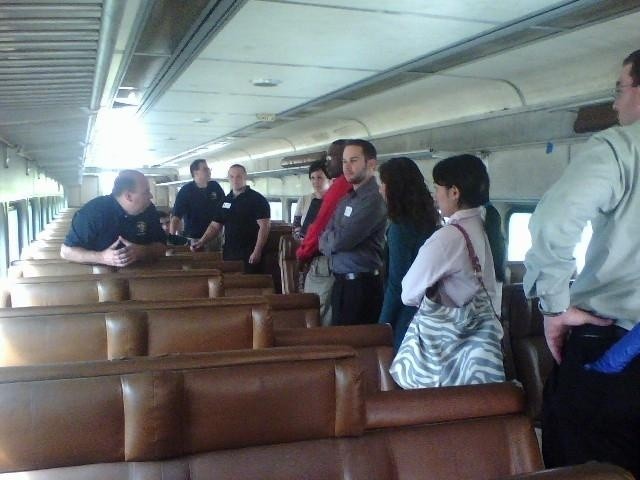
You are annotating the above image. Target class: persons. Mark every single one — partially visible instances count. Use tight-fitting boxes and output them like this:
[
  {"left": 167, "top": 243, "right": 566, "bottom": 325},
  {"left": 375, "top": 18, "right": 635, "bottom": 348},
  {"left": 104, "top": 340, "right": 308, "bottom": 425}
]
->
[
  {"left": 169, "top": 158, "right": 227, "bottom": 254},
  {"left": 522, "top": 49, "right": 639, "bottom": 479},
  {"left": 376, "top": 156, "right": 445, "bottom": 366},
  {"left": 291, "top": 138, "right": 391, "bottom": 328},
  {"left": 399, "top": 153, "right": 507, "bottom": 358},
  {"left": 190, "top": 164, "right": 271, "bottom": 274},
  {"left": 59, "top": 169, "right": 167, "bottom": 268},
  {"left": 155, "top": 209, "right": 170, "bottom": 238}
]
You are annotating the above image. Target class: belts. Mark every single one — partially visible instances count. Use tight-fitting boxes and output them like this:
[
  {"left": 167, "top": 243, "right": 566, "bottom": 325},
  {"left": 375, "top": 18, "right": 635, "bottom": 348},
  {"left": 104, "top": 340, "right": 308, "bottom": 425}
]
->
[{"left": 333, "top": 270, "right": 380, "bottom": 280}]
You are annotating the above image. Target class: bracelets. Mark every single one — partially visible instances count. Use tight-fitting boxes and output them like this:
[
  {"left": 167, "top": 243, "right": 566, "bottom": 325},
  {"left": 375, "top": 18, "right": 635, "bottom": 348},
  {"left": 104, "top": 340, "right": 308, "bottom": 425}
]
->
[{"left": 537, "top": 300, "right": 568, "bottom": 318}]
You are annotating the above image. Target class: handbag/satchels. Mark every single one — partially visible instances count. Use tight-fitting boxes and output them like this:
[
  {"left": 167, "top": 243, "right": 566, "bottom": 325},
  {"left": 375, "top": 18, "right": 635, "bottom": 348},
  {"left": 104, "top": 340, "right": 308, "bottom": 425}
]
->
[{"left": 389, "top": 224, "right": 505, "bottom": 390}]
